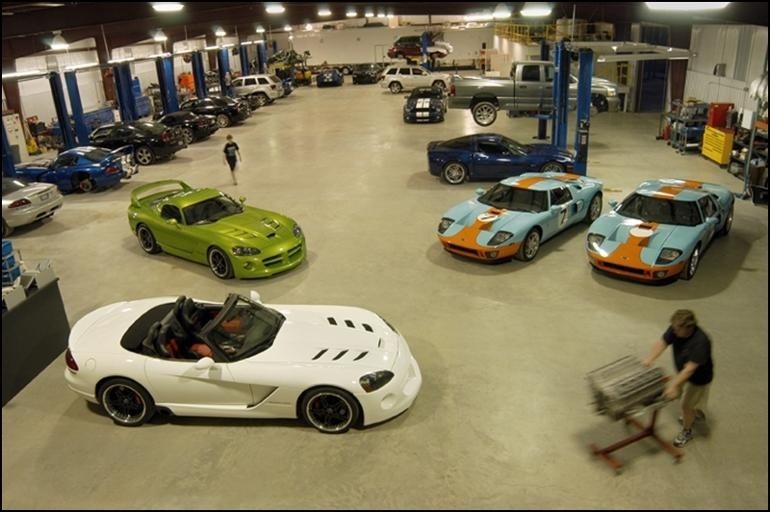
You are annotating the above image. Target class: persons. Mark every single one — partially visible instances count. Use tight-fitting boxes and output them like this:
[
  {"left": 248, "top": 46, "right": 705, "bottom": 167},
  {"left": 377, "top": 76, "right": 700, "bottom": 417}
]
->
[
  {"left": 249, "top": 58, "right": 256, "bottom": 73},
  {"left": 224, "top": 71, "right": 234, "bottom": 98},
  {"left": 223, "top": 134, "right": 243, "bottom": 186},
  {"left": 642, "top": 308, "right": 714, "bottom": 447}
]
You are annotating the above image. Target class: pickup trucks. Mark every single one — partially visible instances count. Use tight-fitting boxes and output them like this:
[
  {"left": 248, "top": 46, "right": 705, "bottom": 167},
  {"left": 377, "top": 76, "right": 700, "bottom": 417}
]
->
[{"left": 450, "top": 60, "right": 621, "bottom": 128}]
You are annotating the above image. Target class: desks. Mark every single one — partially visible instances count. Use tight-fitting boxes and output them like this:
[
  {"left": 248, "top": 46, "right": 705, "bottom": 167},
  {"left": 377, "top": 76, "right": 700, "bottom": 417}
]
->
[{"left": 1, "top": 277, "right": 70, "bottom": 407}]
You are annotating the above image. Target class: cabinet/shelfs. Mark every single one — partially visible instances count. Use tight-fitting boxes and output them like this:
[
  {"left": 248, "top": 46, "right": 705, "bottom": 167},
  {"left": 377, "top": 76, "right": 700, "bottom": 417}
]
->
[
  {"left": 0, "top": 248, "right": 26, "bottom": 287},
  {"left": 727, "top": 123, "right": 768, "bottom": 183}
]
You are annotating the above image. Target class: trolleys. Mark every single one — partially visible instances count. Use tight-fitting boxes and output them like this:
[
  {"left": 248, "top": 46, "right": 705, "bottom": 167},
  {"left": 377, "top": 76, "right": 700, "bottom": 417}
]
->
[{"left": 591, "top": 377, "right": 683, "bottom": 475}]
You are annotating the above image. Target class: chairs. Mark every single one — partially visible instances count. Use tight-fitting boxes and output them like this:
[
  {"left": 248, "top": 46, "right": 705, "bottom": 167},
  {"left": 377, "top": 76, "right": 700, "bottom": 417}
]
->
[
  {"left": 147, "top": 323, "right": 194, "bottom": 359},
  {"left": 174, "top": 294, "right": 212, "bottom": 333}
]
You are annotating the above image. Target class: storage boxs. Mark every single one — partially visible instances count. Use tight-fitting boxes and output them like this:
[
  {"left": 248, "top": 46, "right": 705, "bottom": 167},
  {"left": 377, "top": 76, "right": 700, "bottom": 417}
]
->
[
  {"left": 1, "top": 282, "right": 27, "bottom": 311},
  {"left": 21, "top": 258, "right": 58, "bottom": 290},
  {"left": 1, "top": 237, "right": 19, "bottom": 282}
]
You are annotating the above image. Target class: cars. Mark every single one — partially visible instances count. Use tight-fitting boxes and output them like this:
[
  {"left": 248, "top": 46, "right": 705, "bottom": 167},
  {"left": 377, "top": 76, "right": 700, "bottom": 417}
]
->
[
  {"left": 382, "top": 65, "right": 450, "bottom": 94},
  {"left": 353, "top": 63, "right": 384, "bottom": 84},
  {"left": 231, "top": 74, "right": 285, "bottom": 108},
  {"left": 276, "top": 71, "right": 294, "bottom": 96},
  {"left": 403, "top": 87, "right": 447, "bottom": 124},
  {"left": 157, "top": 111, "right": 218, "bottom": 145},
  {"left": 180, "top": 96, "right": 250, "bottom": 127},
  {"left": 94, "top": 121, "right": 189, "bottom": 165},
  {"left": 388, "top": 35, "right": 453, "bottom": 60}
]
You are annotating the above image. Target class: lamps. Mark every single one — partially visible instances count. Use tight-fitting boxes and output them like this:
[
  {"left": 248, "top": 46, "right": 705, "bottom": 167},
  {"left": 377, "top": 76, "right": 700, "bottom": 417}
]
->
[
  {"left": 317, "top": 5, "right": 332, "bottom": 16},
  {"left": 387, "top": 10, "right": 394, "bottom": 18},
  {"left": 257, "top": 25, "right": 265, "bottom": 33},
  {"left": 154, "top": 28, "right": 168, "bottom": 42},
  {"left": 284, "top": 24, "right": 292, "bottom": 31},
  {"left": 346, "top": 6, "right": 357, "bottom": 18},
  {"left": 215, "top": 27, "right": 227, "bottom": 37},
  {"left": 464, "top": 1, "right": 553, "bottom": 22},
  {"left": 377, "top": 8, "right": 387, "bottom": 18},
  {"left": 51, "top": 30, "right": 70, "bottom": 51},
  {"left": 365, "top": 6, "right": 374, "bottom": 18}
]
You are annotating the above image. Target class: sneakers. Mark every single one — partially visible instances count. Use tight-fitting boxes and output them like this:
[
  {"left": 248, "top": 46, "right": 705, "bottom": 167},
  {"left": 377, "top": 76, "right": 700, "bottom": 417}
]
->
[
  {"left": 673, "top": 429, "right": 694, "bottom": 448},
  {"left": 678, "top": 410, "right": 706, "bottom": 425}
]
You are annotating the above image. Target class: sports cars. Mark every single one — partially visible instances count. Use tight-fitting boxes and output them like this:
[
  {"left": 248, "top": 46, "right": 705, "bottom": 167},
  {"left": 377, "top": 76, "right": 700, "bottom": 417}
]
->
[
  {"left": 436, "top": 171, "right": 602, "bottom": 267},
  {"left": 316, "top": 68, "right": 344, "bottom": 88},
  {"left": 14, "top": 146, "right": 128, "bottom": 194},
  {"left": 126, "top": 178, "right": 306, "bottom": 278},
  {"left": 584, "top": 177, "right": 734, "bottom": 283},
  {"left": 428, "top": 133, "right": 574, "bottom": 186},
  {"left": 64, "top": 290, "right": 422, "bottom": 434},
  {"left": 1, "top": 177, "right": 65, "bottom": 238}
]
